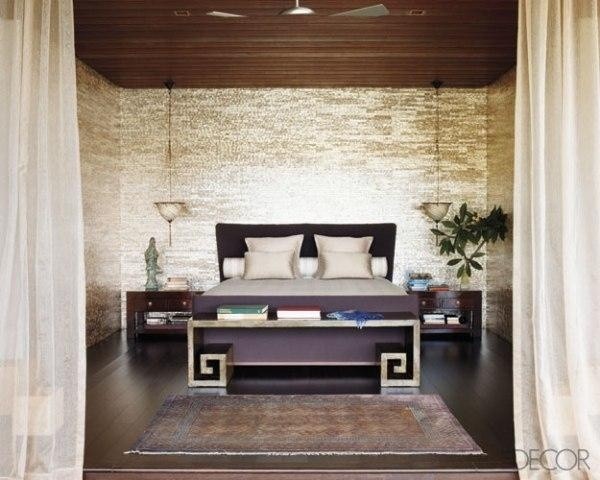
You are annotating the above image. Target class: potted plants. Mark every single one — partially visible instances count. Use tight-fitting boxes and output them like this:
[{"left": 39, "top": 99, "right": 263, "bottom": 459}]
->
[{"left": 429, "top": 203, "right": 510, "bottom": 290}]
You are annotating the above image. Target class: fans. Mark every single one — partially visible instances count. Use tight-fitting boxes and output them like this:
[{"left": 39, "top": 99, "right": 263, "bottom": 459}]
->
[{"left": 205, "top": 0, "right": 390, "bottom": 19}]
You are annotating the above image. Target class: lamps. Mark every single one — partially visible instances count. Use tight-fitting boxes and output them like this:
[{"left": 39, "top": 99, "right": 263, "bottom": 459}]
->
[
  {"left": 422, "top": 80, "right": 452, "bottom": 230},
  {"left": 155, "top": 80, "right": 185, "bottom": 245}
]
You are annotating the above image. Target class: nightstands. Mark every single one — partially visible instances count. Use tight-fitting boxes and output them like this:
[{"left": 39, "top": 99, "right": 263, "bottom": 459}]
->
[
  {"left": 126, "top": 291, "right": 205, "bottom": 340},
  {"left": 409, "top": 290, "right": 482, "bottom": 341}
]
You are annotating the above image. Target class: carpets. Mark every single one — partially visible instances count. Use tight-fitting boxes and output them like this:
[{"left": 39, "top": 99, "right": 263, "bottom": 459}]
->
[{"left": 123, "top": 394, "right": 488, "bottom": 455}]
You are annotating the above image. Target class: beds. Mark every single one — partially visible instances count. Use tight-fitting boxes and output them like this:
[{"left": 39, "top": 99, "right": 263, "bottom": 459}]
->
[{"left": 187, "top": 223, "right": 420, "bottom": 389}]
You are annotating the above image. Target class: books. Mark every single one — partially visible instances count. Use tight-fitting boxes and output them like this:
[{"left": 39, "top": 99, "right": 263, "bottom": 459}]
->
[
  {"left": 407, "top": 272, "right": 433, "bottom": 293},
  {"left": 428, "top": 283, "right": 449, "bottom": 292},
  {"left": 216, "top": 302, "right": 269, "bottom": 314},
  {"left": 445, "top": 314, "right": 459, "bottom": 321},
  {"left": 276, "top": 303, "right": 321, "bottom": 320},
  {"left": 418, "top": 310, "right": 446, "bottom": 320},
  {"left": 446, "top": 320, "right": 461, "bottom": 325},
  {"left": 144, "top": 312, "right": 190, "bottom": 326},
  {"left": 216, "top": 311, "right": 270, "bottom": 321},
  {"left": 420, "top": 319, "right": 447, "bottom": 325}
]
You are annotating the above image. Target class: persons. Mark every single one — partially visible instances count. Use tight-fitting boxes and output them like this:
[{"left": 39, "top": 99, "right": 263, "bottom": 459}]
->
[{"left": 144, "top": 237, "right": 163, "bottom": 291}]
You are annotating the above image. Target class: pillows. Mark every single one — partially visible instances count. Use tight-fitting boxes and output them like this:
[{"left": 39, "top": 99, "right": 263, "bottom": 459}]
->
[{"left": 222, "top": 234, "right": 389, "bottom": 280}]
